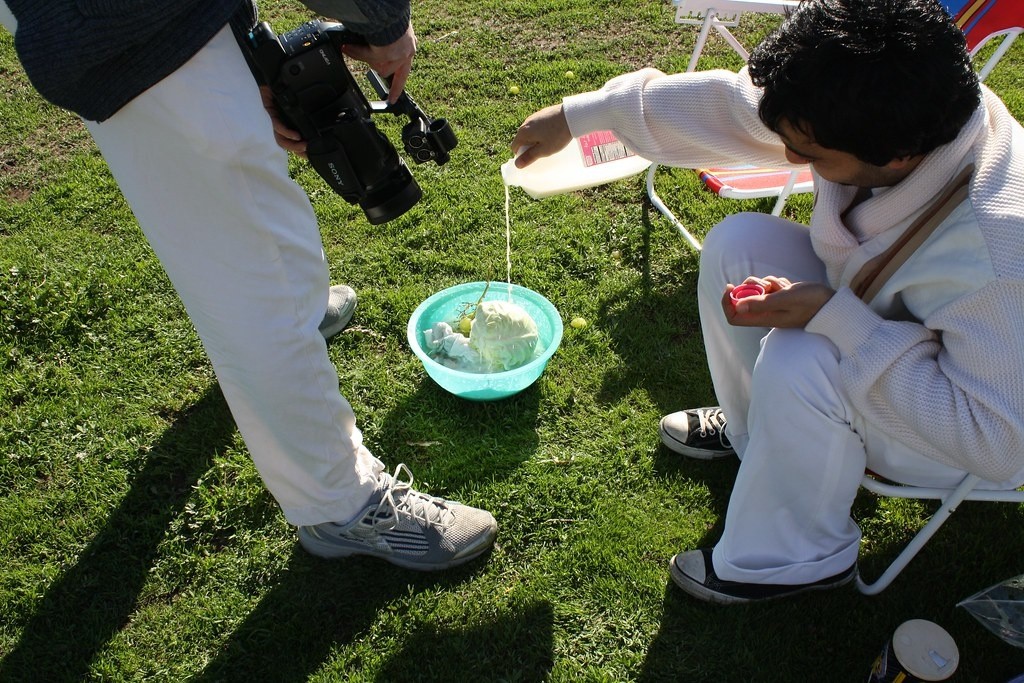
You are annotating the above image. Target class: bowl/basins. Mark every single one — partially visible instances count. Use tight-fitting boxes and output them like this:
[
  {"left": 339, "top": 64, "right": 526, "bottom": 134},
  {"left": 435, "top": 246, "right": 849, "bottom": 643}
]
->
[{"left": 407, "top": 281, "right": 564, "bottom": 400}]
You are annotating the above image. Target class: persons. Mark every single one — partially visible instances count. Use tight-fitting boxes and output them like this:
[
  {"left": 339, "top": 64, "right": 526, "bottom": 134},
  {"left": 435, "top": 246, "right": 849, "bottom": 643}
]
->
[
  {"left": 510, "top": 0, "right": 1024, "bottom": 604},
  {"left": 0, "top": 0, "right": 498, "bottom": 571}
]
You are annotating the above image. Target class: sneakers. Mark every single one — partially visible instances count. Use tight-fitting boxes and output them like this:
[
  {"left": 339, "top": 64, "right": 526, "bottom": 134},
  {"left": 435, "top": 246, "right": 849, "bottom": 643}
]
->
[
  {"left": 299, "top": 463, "right": 498, "bottom": 571},
  {"left": 671, "top": 548, "right": 859, "bottom": 605},
  {"left": 659, "top": 407, "right": 737, "bottom": 458},
  {"left": 317, "top": 284, "right": 358, "bottom": 339}
]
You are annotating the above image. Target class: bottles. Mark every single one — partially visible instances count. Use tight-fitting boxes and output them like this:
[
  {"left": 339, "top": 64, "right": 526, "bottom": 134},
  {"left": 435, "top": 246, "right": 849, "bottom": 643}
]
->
[{"left": 501, "top": 129, "right": 654, "bottom": 200}]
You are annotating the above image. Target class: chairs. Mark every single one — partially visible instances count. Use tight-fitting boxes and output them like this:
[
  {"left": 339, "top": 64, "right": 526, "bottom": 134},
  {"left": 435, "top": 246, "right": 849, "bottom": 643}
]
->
[
  {"left": 647, "top": 1, "right": 1023, "bottom": 252},
  {"left": 854, "top": 473, "right": 1024, "bottom": 596}
]
